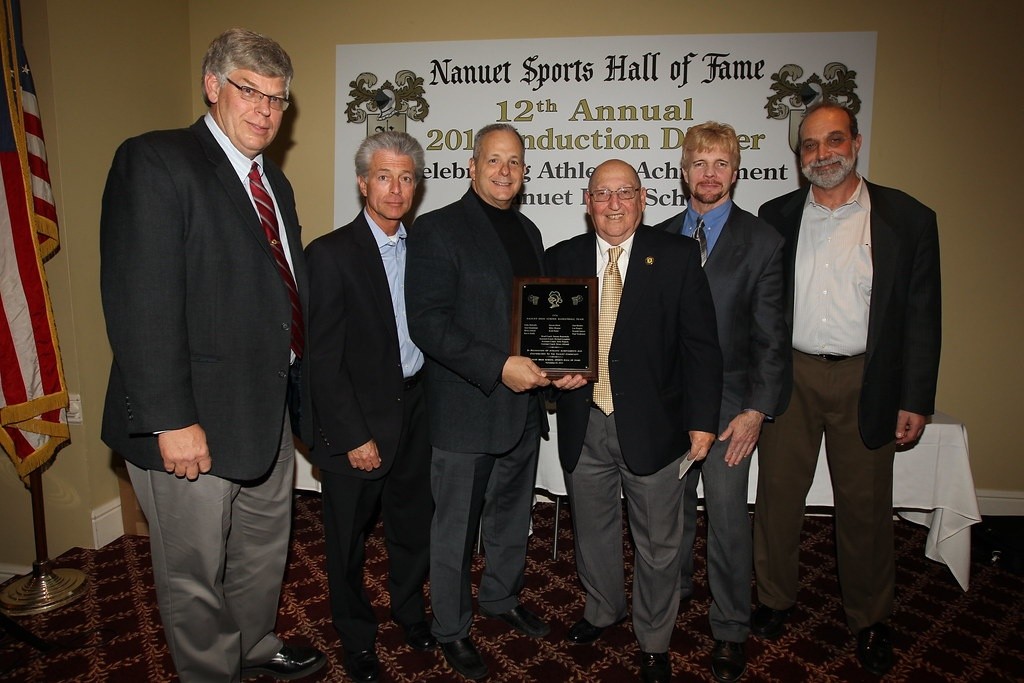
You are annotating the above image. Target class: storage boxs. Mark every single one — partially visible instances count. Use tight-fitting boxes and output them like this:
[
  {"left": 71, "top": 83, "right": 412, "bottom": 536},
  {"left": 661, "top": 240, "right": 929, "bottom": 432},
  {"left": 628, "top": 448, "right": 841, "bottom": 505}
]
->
[{"left": 116, "top": 466, "right": 149, "bottom": 537}]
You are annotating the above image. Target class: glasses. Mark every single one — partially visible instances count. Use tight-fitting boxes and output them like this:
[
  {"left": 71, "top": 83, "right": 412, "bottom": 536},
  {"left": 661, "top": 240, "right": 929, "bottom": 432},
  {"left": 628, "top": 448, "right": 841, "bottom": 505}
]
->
[
  {"left": 589, "top": 186, "right": 640, "bottom": 202},
  {"left": 225, "top": 77, "right": 293, "bottom": 112}
]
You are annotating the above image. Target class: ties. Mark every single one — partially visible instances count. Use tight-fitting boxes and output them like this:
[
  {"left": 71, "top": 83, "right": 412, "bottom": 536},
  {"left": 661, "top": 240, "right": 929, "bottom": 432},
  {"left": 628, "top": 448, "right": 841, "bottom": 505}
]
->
[
  {"left": 592, "top": 245, "right": 624, "bottom": 417},
  {"left": 246, "top": 161, "right": 305, "bottom": 361},
  {"left": 691, "top": 216, "right": 707, "bottom": 268}
]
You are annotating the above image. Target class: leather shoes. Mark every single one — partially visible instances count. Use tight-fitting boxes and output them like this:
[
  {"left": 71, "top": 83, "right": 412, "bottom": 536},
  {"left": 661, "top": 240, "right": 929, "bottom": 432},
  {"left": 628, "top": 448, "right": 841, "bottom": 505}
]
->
[
  {"left": 709, "top": 639, "right": 748, "bottom": 683},
  {"left": 342, "top": 643, "right": 384, "bottom": 683},
  {"left": 566, "top": 610, "right": 628, "bottom": 645},
  {"left": 488, "top": 601, "right": 552, "bottom": 638},
  {"left": 855, "top": 622, "right": 896, "bottom": 674},
  {"left": 400, "top": 619, "right": 435, "bottom": 651},
  {"left": 752, "top": 607, "right": 785, "bottom": 641},
  {"left": 436, "top": 635, "right": 491, "bottom": 680},
  {"left": 240, "top": 643, "right": 327, "bottom": 680},
  {"left": 637, "top": 651, "right": 673, "bottom": 683}
]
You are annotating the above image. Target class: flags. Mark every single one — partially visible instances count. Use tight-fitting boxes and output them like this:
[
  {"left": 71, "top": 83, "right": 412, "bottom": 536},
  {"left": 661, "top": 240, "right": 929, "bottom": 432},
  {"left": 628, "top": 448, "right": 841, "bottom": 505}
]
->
[{"left": 0, "top": 0, "right": 72, "bottom": 490}]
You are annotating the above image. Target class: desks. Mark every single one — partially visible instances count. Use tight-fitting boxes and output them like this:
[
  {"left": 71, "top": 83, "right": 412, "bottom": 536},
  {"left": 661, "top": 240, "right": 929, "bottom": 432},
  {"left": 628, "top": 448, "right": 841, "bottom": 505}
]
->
[{"left": 534, "top": 413, "right": 982, "bottom": 593}]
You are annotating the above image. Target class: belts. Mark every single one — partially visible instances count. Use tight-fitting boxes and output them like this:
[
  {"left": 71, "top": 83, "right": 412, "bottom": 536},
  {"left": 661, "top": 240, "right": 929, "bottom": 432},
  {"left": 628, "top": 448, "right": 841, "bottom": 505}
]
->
[
  {"left": 812, "top": 353, "right": 850, "bottom": 362},
  {"left": 404, "top": 376, "right": 421, "bottom": 393}
]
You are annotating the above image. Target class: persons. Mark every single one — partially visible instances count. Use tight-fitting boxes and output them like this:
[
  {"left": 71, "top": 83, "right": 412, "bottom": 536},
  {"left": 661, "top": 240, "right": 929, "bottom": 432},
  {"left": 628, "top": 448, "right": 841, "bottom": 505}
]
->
[
  {"left": 650, "top": 120, "right": 789, "bottom": 679},
  {"left": 751, "top": 103, "right": 940, "bottom": 671},
  {"left": 538, "top": 156, "right": 719, "bottom": 683},
  {"left": 100, "top": 26, "right": 329, "bottom": 681},
  {"left": 404, "top": 124, "right": 588, "bottom": 680},
  {"left": 301, "top": 129, "right": 438, "bottom": 681}
]
